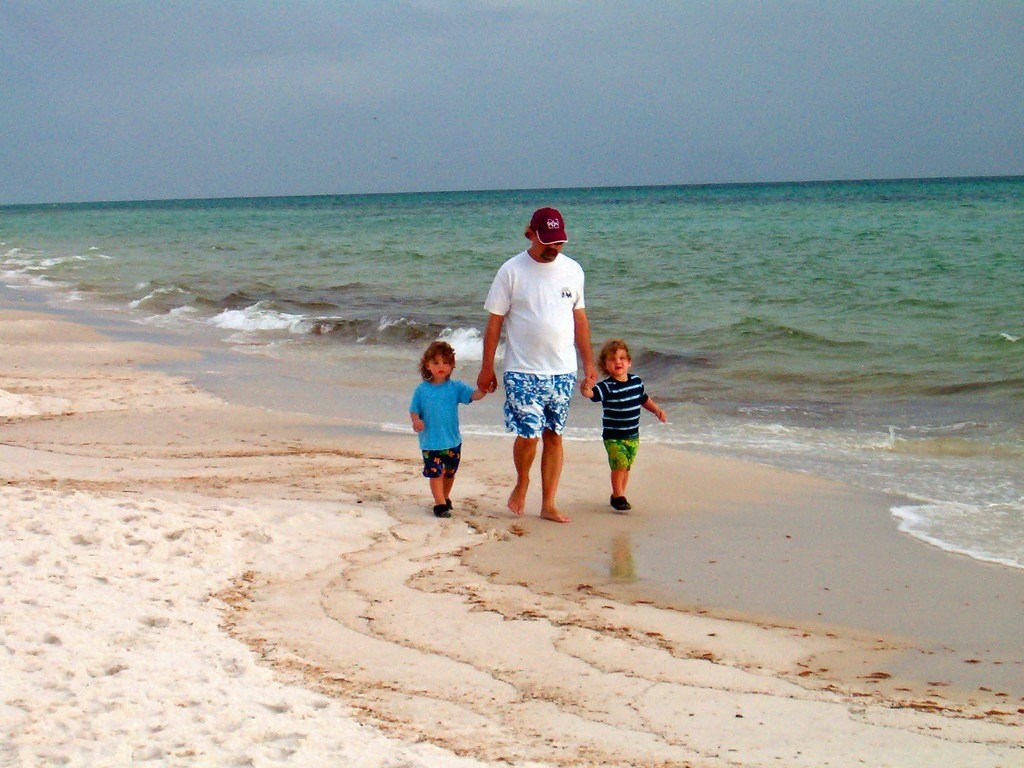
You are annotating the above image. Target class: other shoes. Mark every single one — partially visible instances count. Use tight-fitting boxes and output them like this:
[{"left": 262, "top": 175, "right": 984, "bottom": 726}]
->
[
  {"left": 433, "top": 497, "right": 454, "bottom": 518},
  {"left": 610, "top": 494, "right": 632, "bottom": 513}
]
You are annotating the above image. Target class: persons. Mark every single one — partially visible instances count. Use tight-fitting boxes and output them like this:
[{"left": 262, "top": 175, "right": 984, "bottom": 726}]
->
[
  {"left": 476, "top": 207, "right": 598, "bottom": 523},
  {"left": 581, "top": 339, "right": 666, "bottom": 512},
  {"left": 409, "top": 341, "right": 497, "bottom": 518}
]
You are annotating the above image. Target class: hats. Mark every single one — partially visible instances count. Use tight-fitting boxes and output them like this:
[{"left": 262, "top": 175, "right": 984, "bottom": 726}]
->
[{"left": 529, "top": 207, "right": 568, "bottom": 245}]
